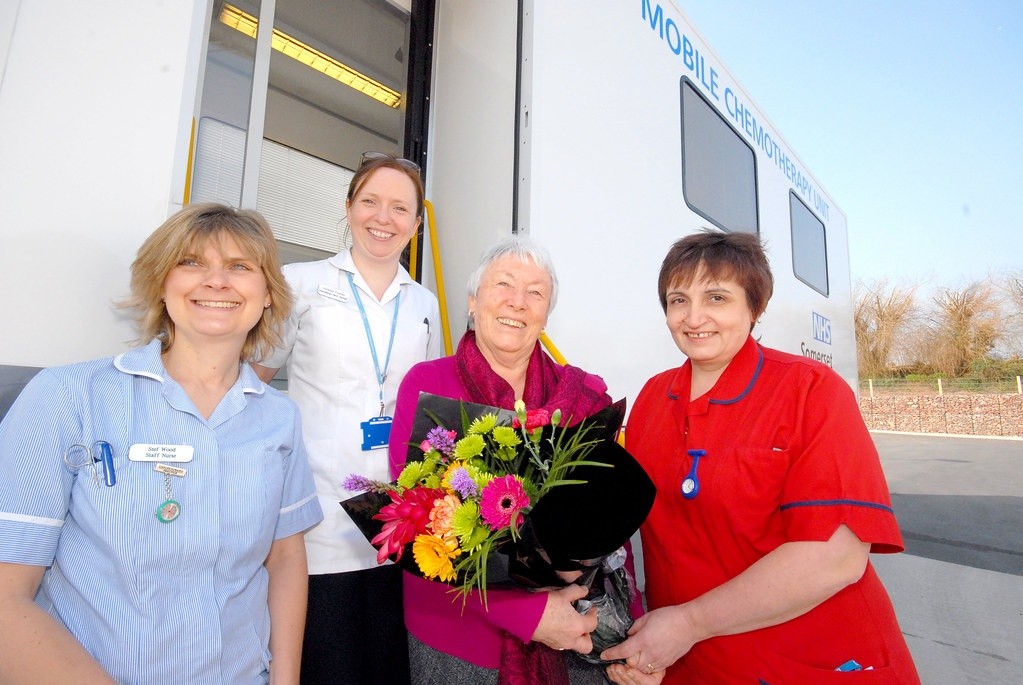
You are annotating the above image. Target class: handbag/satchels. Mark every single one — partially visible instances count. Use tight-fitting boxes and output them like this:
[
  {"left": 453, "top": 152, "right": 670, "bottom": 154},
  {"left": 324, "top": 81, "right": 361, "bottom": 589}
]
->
[{"left": 564, "top": 566, "right": 634, "bottom": 685}]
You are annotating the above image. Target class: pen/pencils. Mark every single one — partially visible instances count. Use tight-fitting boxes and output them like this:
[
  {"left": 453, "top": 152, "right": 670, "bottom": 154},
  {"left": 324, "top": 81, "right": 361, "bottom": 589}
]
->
[
  {"left": 423, "top": 317, "right": 429, "bottom": 334},
  {"left": 98, "top": 443, "right": 117, "bottom": 486}
]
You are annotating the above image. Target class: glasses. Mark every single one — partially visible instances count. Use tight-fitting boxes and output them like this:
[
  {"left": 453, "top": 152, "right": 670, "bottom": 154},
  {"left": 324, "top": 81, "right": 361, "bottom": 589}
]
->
[{"left": 358, "top": 151, "right": 421, "bottom": 178}]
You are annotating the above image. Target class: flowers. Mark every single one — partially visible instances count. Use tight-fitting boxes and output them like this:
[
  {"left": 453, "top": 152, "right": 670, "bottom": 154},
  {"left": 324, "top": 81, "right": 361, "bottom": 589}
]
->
[{"left": 340, "top": 397, "right": 639, "bottom": 665}]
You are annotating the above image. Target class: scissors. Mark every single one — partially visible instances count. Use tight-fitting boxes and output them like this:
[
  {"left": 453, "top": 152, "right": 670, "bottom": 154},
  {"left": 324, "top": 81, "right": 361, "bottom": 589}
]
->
[{"left": 63, "top": 440, "right": 114, "bottom": 490}]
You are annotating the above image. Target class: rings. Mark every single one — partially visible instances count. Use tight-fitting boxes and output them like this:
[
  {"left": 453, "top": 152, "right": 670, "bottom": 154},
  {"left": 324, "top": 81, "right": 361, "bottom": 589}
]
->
[
  {"left": 648, "top": 664, "right": 655, "bottom": 672},
  {"left": 559, "top": 648, "right": 565, "bottom": 651}
]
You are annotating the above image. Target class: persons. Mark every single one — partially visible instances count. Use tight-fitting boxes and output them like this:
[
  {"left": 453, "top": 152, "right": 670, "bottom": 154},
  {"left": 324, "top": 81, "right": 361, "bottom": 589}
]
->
[
  {"left": 0, "top": 200, "right": 323, "bottom": 684},
  {"left": 248, "top": 151, "right": 443, "bottom": 685},
  {"left": 387, "top": 235, "right": 647, "bottom": 685},
  {"left": 598, "top": 230, "right": 922, "bottom": 685}
]
